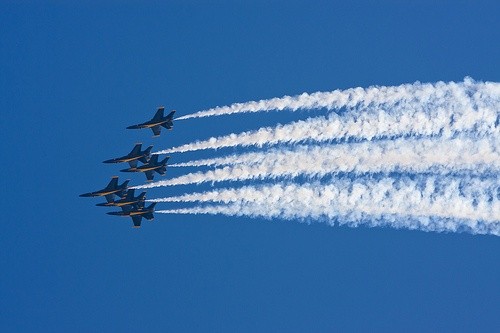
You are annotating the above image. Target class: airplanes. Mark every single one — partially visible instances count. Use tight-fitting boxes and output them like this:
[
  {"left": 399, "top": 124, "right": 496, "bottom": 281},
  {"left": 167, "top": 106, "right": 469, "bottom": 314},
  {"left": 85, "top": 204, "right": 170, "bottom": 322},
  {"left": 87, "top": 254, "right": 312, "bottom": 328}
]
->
[
  {"left": 95, "top": 188, "right": 147, "bottom": 216},
  {"left": 78, "top": 176, "right": 131, "bottom": 203},
  {"left": 125, "top": 106, "right": 176, "bottom": 137},
  {"left": 106, "top": 200, "right": 156, "bottom": 228},
  {"left": 102, "top": 143, "right": 153, "bottom": 169},
  {"left": 120, "top": 154, "right": 170, "bottom": 182}
]
